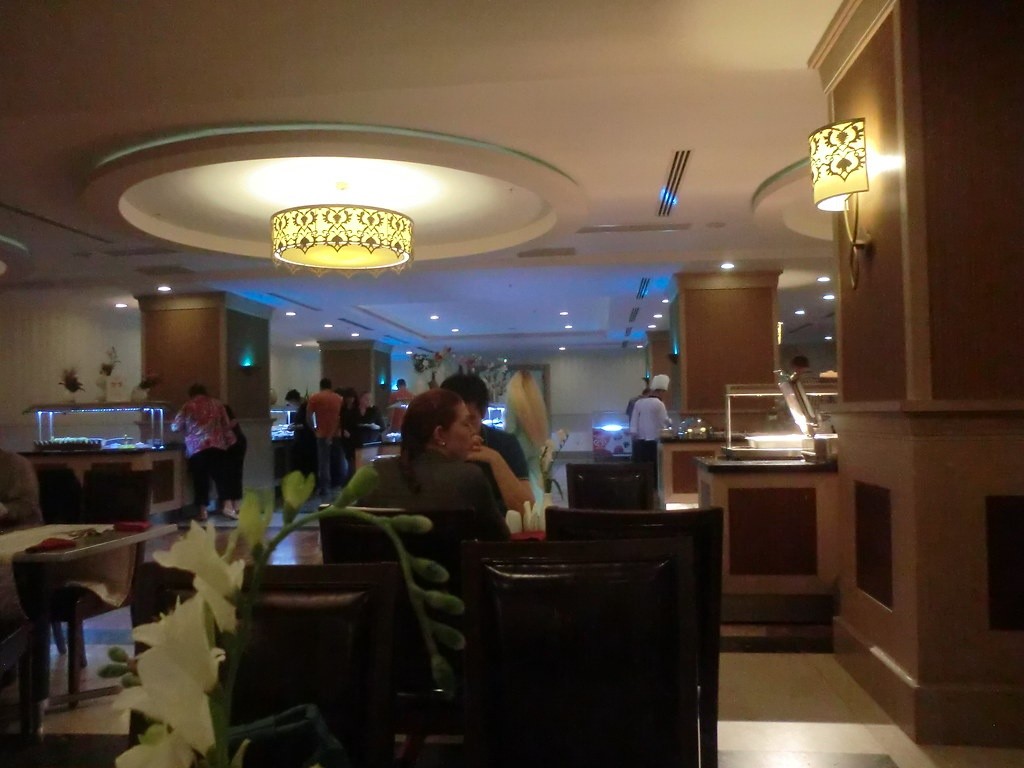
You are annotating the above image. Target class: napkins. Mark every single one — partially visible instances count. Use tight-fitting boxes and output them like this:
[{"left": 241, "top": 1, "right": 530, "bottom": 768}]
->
[
  {"left": 114, "top": 520, "right": 152, "bottom": 533},
  {"left": 25, "top": 536, "right": 77, "bottom": 553},
  {"left": 512, "top": 532, "right": 547, "bottom": 543}
]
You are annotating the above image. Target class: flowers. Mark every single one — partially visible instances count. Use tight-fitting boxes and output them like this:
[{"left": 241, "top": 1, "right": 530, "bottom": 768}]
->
[
  {"left": 535, "top": 428, "right": 570, "bottom": 501},
  {"left": 97, "top": 464, "right": 466, "bottom": 768},
  {"left": 410, "top": 346, "right": 510, "bottom": 396}
]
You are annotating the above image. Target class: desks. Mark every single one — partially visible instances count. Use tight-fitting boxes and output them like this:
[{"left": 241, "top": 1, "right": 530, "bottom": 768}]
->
[{"left": 0, "top": 520, "right": 179, "bottom": 720}]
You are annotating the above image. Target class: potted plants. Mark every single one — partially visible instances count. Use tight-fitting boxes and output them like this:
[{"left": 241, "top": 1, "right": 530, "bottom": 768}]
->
[
  {"left": 56, "top": 370, "right": 86, "bottom": 394},
  {"left": 100, "top": 346, "right": 120, "bottom": 377}
]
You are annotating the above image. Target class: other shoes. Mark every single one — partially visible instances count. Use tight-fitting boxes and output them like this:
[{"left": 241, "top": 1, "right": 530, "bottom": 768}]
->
[
  {"left": 195, "top": 510, "right": 208, "bottom": 520},
  {"left": 223, "top": 508, "right": 239, "bottom": 519}
]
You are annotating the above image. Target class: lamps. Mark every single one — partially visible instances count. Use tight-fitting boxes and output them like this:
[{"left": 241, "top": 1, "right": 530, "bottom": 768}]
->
[
  {"left": 808, "top": 118, "right": 873, "bottom": 291},
  {"left": 270, "top": 204, "right": 415, "bottom": 279}
]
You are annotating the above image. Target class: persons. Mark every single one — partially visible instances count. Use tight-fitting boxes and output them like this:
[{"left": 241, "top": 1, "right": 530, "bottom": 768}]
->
[
  {"left": 626, "top": 386, "right": 652, "bottom": 425},
  {"left": 387, "top": 379, "right": 418, "bottom": 438},
  {"left": 629, "top": 374, "right": 676, "bottom": 491},
  {"left": 353, "top": 388, "right": 518, "bottom": 548},
  {"left": 305, "top": 378, "right": 383, "bottom": 499},
  {"left": 285, "top": 388, "right": 316, "bottom": 496},
  {"left": 423, "top": 374, "right": 535, "bottom": 534},
  {"left": 171, "top": 383, "right": 238, "bottom": 521},
  {"left": 787, "top": 355, "right": 818, "bottom": 382},
  {"left": 206, "top": 401, "right": 247, "bottom": 514},
  {"left": 0, "top": 440, "right": 62, "bottom": 711}
]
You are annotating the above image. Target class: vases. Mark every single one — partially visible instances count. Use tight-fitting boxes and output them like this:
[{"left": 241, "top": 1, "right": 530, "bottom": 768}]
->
[
  {"left": 537, "top": 491, "right": 555, "bottom": 532},
  {"left": 428, "top": 370, "right": 440, "bottom": 389}
]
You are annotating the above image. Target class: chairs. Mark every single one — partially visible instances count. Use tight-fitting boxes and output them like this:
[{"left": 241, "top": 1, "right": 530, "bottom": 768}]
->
[{"left": 128, "top": 460, "right": 723, "bottom": 768}]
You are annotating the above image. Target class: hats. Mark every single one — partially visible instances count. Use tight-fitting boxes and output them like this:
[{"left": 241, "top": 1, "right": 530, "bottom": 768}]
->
[{"left": 651, "top": 374, "right": 670, "bottom": 392}]
